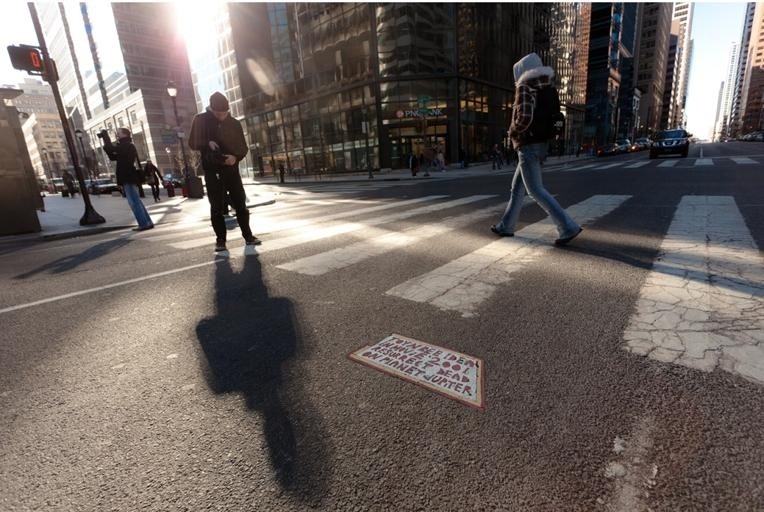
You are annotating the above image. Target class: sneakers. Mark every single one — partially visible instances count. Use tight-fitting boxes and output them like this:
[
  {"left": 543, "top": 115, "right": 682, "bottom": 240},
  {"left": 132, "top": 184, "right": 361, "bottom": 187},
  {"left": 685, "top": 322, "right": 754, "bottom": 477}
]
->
[
  {"left": 555, "top": 227, "right": 583, "bottom": 245},
  {"left": 132, "top": 224, "right": 154, "bottom": 231},
  {"left": 489, "top": 224, "right": 515, "bottom": 236},
  {"left": 154, "top": 196, "right": 161, "bottom": 202},
  {"left": 246, "top": 236, "right": 261, "bottom": 245},
  {"left": 215, "top": 239, "right": 226, "bottom": 250}
]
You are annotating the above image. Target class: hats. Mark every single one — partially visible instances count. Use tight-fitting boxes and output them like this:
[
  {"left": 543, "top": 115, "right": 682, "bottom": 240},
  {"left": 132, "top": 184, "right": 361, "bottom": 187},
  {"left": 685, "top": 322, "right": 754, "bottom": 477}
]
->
[{"left": 210, "top": 92, "right": 229, "bottom": 112}]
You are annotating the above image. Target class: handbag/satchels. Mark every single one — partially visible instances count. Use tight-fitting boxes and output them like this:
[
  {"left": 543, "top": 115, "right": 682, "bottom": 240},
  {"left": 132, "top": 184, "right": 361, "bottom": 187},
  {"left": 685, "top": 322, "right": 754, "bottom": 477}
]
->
[{"left": 136, "top": 167, "right": 146, "bottom": 184}]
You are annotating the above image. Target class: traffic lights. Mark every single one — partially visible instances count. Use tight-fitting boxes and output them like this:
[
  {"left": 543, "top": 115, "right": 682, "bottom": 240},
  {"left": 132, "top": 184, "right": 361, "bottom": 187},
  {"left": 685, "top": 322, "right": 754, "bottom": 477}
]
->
[{"left": 8, "top": 45, "right": 45, "bottom": 73}]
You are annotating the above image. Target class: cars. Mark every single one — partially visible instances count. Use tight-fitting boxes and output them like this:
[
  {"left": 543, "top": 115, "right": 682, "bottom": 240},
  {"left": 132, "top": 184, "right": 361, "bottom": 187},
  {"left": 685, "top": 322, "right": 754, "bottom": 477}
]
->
[
  {"left": 719, "top": 131, "right": 763, "bottom": 144},
  {"left": 47, "top": 174, "right": 117, "bottom": 196},
  {"left": 595, "top": 126, "right": 693, "bottom": 159}
]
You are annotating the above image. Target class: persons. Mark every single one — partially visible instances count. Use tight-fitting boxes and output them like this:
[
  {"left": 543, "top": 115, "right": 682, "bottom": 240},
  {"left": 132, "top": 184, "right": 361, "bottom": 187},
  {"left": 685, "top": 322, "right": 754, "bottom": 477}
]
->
[
  {"left": 277, "top": 163, "right": 284, "bottom": 184},
  {"left": 100, "top": 127, "right": 152, "bottom": 231},
  {"left": 408, "top": 152, "right": 416, "bottom": 179},
  {"left": 186, "top": 91, "right": 261, "bottom": 250},
  {"left": 62, "top": 168, "right": 75, "bottom": 198},
  {"left": 143, "top": 159, "right": 163, "bottom": 203},
  {"left": 490, "top": 144, "right": 503, "bottom": 171},
  {"left": 487, "top": 53, "right": 583, "bottom": 243}
]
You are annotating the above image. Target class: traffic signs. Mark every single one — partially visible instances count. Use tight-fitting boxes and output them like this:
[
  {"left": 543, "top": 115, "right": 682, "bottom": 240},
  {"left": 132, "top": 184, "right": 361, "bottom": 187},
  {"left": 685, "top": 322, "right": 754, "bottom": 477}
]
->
[
  {"left": 416, "top": 109, "right": 432, "bottom": 113},
  {"left": 418, "top": 95, "right": 434, "bottom": 101}
]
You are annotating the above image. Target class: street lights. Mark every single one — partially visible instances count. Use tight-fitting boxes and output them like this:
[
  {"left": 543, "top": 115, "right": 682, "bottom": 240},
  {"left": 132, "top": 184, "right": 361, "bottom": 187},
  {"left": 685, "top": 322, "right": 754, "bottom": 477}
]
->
[
  {"left": 162, "top": 81, "right": 189, "bottom": 177},
  {"left": 359, "top": 106, "right": 373, "bottom": 180},
  {"left": 73, "top": 129, "right": 94, "bottom": 189}
]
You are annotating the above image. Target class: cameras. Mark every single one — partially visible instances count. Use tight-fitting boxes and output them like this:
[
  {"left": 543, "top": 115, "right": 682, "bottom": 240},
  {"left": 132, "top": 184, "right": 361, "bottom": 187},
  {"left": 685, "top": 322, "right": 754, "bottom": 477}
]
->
[{"left": 97, "top": 129, "right": 106, "bottom": 139}]
